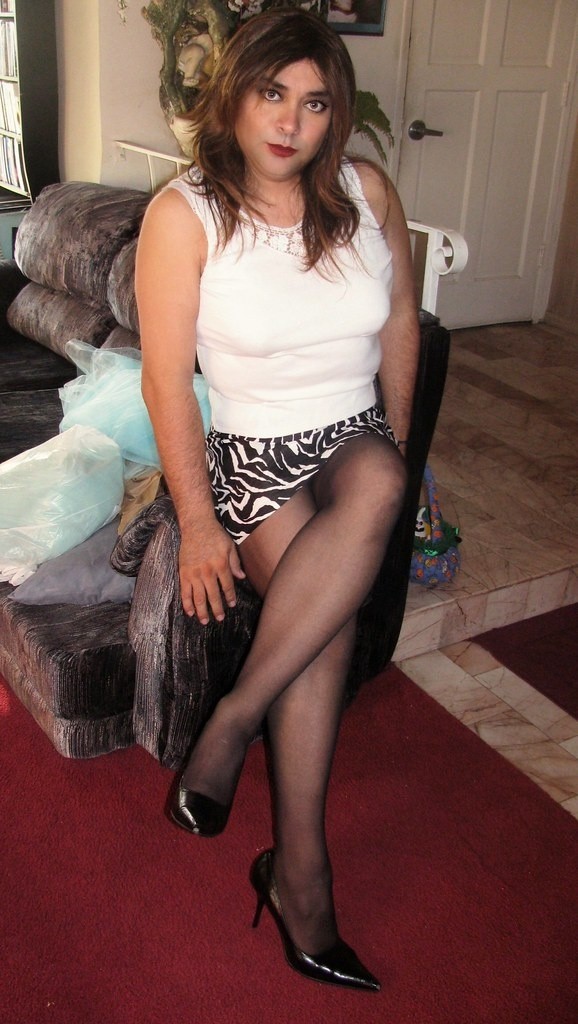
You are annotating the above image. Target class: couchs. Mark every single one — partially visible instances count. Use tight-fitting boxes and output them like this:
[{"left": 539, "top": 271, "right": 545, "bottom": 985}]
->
[{"left": 0, "top": 180, "right": 467, "bottom": 772}]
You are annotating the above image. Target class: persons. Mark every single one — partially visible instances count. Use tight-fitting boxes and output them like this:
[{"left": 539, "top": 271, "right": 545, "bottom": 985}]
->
[{"left": 134, "top": 7, "right": 420, "bottom": 994}]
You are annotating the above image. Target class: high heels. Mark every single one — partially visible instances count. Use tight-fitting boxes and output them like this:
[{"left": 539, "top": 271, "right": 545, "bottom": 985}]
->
[
  {"left": 170, "top": 713, "right": 248, "bottom": 837},
  {"left": 249, "top": 848, "right": 381, "bottom": 992}
]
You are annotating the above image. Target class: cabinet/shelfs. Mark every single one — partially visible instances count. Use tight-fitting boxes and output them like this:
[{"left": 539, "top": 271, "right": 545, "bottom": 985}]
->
[{"left": 0, "top": 0, "right": 61, "bottom": 210}]
